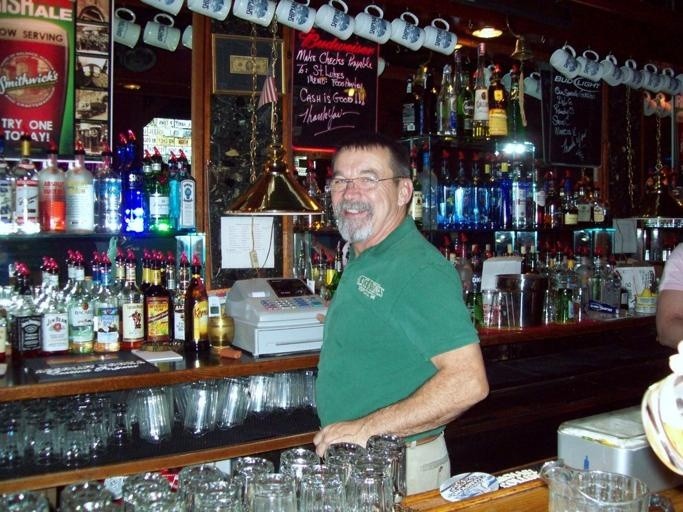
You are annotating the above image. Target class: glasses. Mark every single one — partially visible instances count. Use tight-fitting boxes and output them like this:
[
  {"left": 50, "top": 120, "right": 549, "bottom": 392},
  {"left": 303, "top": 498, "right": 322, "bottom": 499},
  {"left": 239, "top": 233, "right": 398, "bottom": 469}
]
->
[{"left": 326, "top": 175, "right": 410, "bottom": 190}]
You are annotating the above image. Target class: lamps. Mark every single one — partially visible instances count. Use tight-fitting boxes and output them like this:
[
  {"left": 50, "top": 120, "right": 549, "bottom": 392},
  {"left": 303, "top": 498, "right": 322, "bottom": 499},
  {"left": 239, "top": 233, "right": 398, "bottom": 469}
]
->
[
  {"left": 468, "top": 7, "right": 503, "bottom": 39},
  {"left": 222, "top": 16, "right": 324, "bottom": 217},
  {"left": 642, "top": 99, "right": 683, "bottom": 228}
]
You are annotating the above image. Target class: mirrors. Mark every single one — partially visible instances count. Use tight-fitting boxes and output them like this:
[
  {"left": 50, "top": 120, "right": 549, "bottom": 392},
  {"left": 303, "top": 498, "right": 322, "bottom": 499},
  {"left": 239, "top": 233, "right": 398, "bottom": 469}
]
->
[
  {"left": 108, "top": 0, "right": 193, "bottom": 182},
  {"left": 212, "top": 19, "right": 285, "bottom": 283}
]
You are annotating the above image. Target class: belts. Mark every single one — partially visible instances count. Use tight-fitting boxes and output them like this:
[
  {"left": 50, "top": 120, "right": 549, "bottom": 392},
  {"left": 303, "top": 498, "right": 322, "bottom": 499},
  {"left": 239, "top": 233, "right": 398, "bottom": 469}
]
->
[{"left": 405, "top": 432, "right": 442, "bottom": 448}]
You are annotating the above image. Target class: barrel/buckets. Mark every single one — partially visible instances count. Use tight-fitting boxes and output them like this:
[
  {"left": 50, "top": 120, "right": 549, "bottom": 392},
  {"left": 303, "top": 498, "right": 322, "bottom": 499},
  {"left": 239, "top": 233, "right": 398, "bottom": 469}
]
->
[{"left": 495, "top": 274, "right": 546, "bottom": 329}]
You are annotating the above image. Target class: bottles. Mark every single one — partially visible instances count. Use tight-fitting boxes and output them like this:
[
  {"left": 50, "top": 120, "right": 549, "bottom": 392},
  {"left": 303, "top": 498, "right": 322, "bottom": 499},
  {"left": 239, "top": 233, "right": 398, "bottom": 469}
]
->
[
  {"left": 0, "top": 248, "right": 235, "bottom": 355},
  {"left": 0, "top": 133, "right": 197, "bottom": 233},
  {"left": 293, "top": 152, "right": 344, "bottom": 297},
  {"left": 408, "top": 139, "right": 611, "bottom": 230},
  {"left": 436, "top": 225, "right": 671, "bottom": 327},
  {"left": 401, "top": 58, "right": 528, "bottom": 139}
]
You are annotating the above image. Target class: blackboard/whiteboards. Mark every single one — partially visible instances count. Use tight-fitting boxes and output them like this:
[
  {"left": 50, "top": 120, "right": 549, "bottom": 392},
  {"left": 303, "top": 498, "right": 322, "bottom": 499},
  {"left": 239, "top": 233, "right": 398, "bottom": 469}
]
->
[
  {"left": 539, "top": 62, "right": 605, "bottom": 168},
  {"left": 289, "top": 28, "right": 380, "bottom": 153}
]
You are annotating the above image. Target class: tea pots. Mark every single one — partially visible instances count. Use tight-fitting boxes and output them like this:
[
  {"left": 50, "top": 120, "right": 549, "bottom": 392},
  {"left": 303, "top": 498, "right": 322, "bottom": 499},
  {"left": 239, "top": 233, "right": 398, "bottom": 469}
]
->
[{"left": 540, "top": 458, "right": 674, "bottom": 511}]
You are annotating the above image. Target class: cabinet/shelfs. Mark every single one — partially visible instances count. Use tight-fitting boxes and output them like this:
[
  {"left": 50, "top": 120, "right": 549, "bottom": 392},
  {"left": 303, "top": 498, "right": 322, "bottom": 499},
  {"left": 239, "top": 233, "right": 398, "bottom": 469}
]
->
[{"left": 0, "top": 229, "right": 672, "bottom": 497}]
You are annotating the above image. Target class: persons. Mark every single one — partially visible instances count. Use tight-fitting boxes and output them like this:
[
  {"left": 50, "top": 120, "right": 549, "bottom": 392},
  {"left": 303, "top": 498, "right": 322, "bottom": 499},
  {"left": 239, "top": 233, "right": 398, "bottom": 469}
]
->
[
  {"left": 314, "top": 132, "right": 489, "bottom": 496},
  {"left": 656, "top": 242, "right": 683, "bottom": 351}
]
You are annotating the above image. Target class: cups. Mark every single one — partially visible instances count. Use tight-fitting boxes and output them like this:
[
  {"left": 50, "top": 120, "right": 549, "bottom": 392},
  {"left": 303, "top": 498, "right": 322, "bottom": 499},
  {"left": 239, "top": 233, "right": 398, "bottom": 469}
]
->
[
  {"left": 354, "top": 5, "right": 392, "bottom": 45},
  {"left": 110, "top": 8, "right": 142, "bottom": 49},
  {"left": 143, "top": 1, "right": 184, "bottom": 16},
  {"left": 274, "top": 1, "right": 317, "bottom": 34},
  {"left": 524, "top": 39, "right": 681, "bottom": 116},
  {"left": 0, "top": 368, "right": 407, "bottom": 512},
  {"left": 314, "top": 0, "right": 354, "bottom": 41},
  {"left": 141, "top": 14, "right": 182, "bottom": 53},
  {"left": 186, "top": 1, "right": 232, "bottom": 21},
  {"left": 390, "top": 12, "right": 425, "bottom": 51},
  {"left": 423, "top": 18, "right": 458, "bottom": 57},
  {"left": 232, "top": 0, "right": 276, "bottom": 28},
  {"left": 181, "top": 24, "right": 192, "bottom": 51}
]
